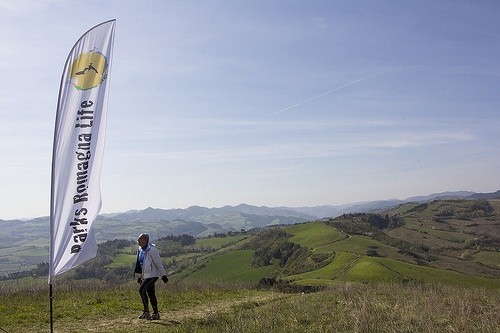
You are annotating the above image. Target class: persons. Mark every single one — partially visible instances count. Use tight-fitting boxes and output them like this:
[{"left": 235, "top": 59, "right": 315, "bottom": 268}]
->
[{"left": 135, "top": 233, "right": 169, "bottom": 320}]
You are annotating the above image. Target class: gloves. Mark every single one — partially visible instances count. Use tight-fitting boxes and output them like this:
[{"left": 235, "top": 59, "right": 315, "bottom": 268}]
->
[
  {"left": 137, "top": 278, "right": 142, "bottom": 283},
  {"left": 162, "top": 275, "right": 168, "bottom": 283}
]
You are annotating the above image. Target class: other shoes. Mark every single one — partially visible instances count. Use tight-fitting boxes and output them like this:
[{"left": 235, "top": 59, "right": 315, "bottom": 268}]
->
[
  {"left": 147, "top": 313, "right": 160, "bottom": 320},
  {"left": 139, "top": 311, "right": 151, "bottom": 319}
]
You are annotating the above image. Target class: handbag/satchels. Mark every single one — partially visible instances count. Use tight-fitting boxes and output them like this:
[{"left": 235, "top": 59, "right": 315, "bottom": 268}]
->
[{"left": 134, "top": 250, "right": 142, "bottom": 273}]
